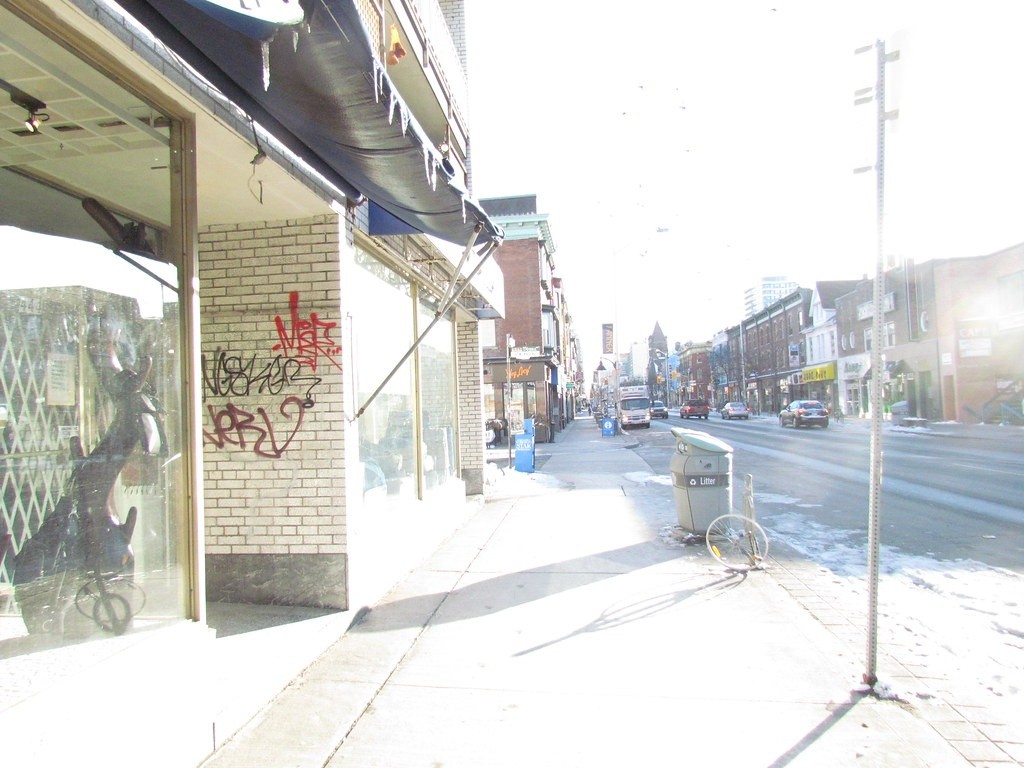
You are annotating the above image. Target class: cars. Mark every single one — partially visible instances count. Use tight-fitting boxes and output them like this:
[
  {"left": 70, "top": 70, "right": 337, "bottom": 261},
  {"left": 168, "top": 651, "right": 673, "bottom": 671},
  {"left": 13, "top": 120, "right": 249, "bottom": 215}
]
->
[
  {"left": 721, "top": 402, "right": 749, "bottom": 420},
  {"left": 650, "top": 401, "right": 668, "bottom": 419},
  {"left": 680, "top": 399, "right": 710, "bottom": 419},
  {"left": 779, "top": 400, "right": 829, "bottom": 428}
]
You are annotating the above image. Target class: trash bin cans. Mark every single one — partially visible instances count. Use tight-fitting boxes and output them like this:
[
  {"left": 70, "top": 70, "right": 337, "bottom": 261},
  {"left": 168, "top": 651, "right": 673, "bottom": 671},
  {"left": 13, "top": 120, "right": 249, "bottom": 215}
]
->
[
  {"left": 667, "top": 427, "right": 734, "bottom": 536},
  {"left": 514, "top": 419, "right": 536, "bottom": 474}
]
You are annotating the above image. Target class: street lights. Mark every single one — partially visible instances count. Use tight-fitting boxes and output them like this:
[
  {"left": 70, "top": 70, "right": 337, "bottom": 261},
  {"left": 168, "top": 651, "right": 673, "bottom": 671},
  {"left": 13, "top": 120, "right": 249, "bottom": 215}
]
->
[{"left": 596, "top": 357, "right": 621, "bottom": 432}]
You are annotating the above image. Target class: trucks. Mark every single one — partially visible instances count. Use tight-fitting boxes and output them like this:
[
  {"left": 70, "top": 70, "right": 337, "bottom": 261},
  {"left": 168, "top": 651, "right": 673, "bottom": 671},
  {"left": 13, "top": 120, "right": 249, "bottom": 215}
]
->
[{"left": 618, "top": 386, "right": 650, "bottom": 428}]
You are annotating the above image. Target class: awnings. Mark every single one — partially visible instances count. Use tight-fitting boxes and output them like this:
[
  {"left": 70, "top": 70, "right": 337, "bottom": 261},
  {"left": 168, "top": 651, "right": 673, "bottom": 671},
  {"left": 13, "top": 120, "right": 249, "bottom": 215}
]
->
[{"left": 127, "top": 0, "right": 505, "bottom": 250}]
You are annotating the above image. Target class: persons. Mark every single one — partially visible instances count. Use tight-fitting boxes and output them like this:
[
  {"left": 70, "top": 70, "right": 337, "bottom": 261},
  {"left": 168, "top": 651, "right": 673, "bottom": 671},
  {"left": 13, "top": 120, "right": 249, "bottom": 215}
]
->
[{"left": 588, "top": 407, "right": 592, "bottom": 416}]
